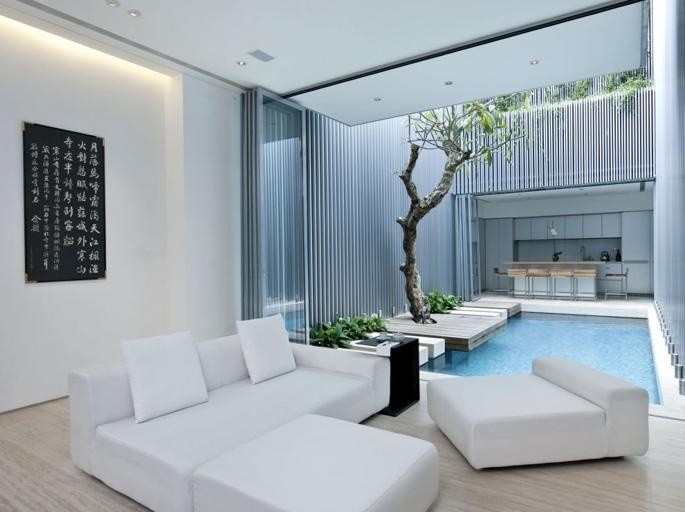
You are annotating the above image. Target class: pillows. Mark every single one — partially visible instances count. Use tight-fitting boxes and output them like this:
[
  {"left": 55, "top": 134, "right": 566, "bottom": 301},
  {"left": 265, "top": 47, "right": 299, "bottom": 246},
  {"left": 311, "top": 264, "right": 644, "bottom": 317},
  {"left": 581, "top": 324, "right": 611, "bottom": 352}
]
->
[
  {"left": 117, "top": 331, "right": 210, "bottom": 426},
  {"left": 235, "top": 313, "right": 297, "bottom": 386}
]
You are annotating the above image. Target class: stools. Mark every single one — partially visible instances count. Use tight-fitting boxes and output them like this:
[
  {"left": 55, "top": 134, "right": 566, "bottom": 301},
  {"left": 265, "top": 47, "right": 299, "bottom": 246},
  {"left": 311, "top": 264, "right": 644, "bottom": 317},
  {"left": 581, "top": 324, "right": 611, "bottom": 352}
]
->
[
  {"left": 491, "top": 268, "right": 511, "bottom": 295},
  {"left": 604, "top": 268, "right": 629, "bottom": 301},
  {"left": 508, "top": 268, "right": 598, "bottom": 302}
]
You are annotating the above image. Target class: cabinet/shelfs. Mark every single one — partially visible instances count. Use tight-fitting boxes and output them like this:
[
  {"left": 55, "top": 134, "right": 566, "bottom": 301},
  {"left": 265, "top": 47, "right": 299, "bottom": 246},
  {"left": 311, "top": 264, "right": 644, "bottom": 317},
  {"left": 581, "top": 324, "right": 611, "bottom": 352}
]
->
[
  {"left": 546, "top": 215, "right": 584, "bottom": 239},
  {"left": 513, "top": 217, "right": 548, "bottom": 240},
  {"left": 484, "top": 219, "right": 513, "bottom": 292},
  {"left": 583, "top": 214, "right": 621, "bottom": 238}
]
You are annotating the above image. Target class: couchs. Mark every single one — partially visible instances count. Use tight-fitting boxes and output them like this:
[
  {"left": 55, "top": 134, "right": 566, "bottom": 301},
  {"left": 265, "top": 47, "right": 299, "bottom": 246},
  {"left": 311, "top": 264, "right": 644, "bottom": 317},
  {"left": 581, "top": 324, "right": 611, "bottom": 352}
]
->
[
  {"left": 65, "top": 341, "right": 391, "bottom": 512},
  {"left": 189, "top": 413, "right": 440, "bottom": 512},
  {"left": 426, "top": 354, "right": 651, "bottom": 472}
]
